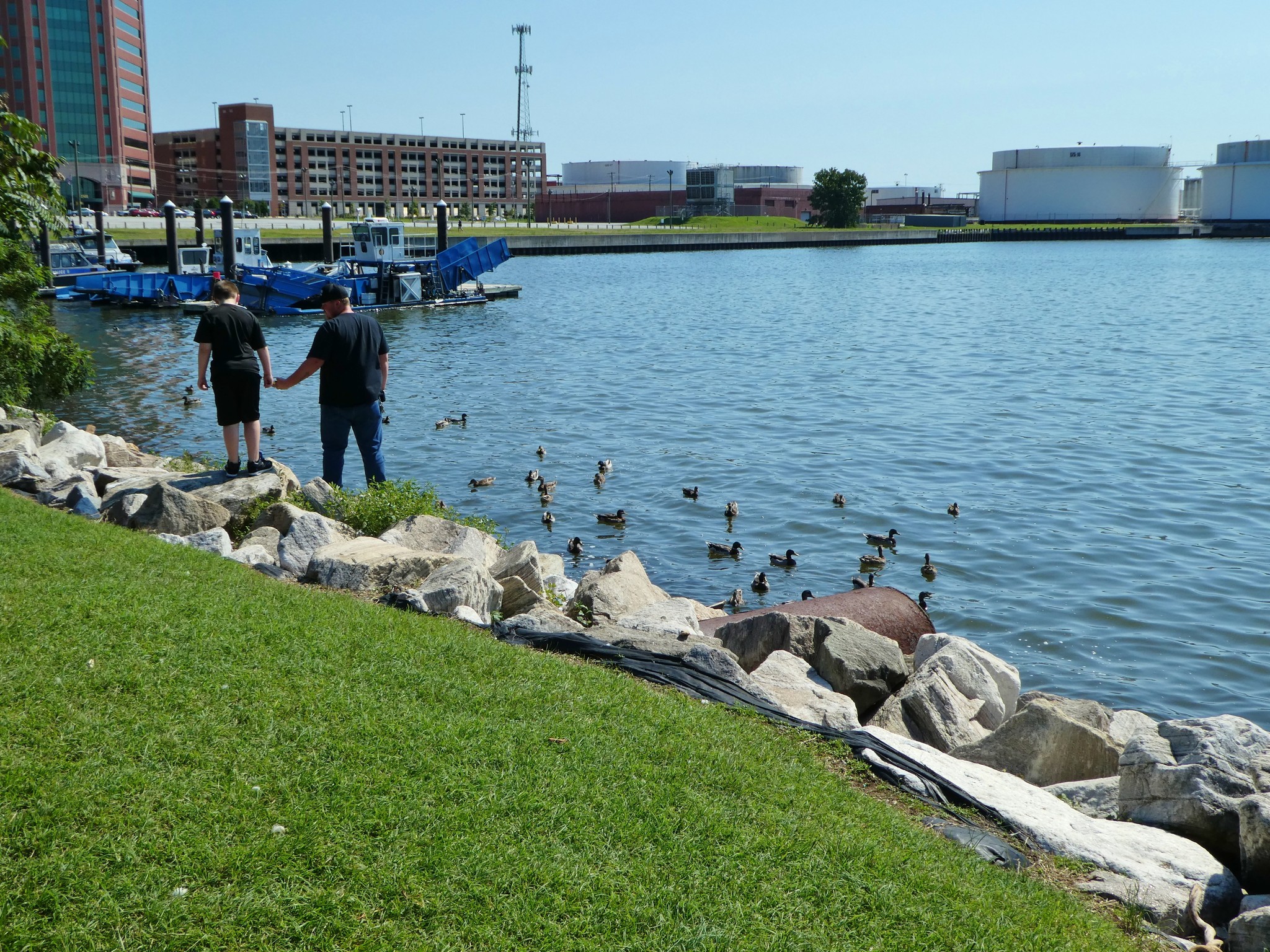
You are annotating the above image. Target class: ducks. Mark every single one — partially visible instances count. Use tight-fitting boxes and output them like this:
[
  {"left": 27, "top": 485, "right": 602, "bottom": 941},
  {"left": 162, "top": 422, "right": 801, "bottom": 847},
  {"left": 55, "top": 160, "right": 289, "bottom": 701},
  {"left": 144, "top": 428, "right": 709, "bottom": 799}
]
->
[
  {"left": 526, "top": 469, "right": 539, "bottom": 481},
  {"left": 435, "top": 413, "right": 469, "bottom": 427},
  {"left": 863, "top": 529, "right": 901, "bottom": 547},
  {"left": 832, "top": 492, "right": 846, "bottom": 504},
  {"left": 535, "top": 445, "right": 545, "bottom": 455},
  {"left": 566, "top": 537, "right": 584, "bottom": 552},
  {"left": 541, "top": 511, "right": 555, "bottom": 523},
  {"left": 723, "top": 501, "right": 738, "bottom": 516},
  {"left": 381, "top": 416, "right": 390, "bottom": 424},
  {"left": 705, "top": 540, "right": 744, "bottom": 554},
  {"left": 262, "top": 424, "right": 276, "bottom": 433},
  {"left": 468, "top": 477, "right": 496, "bottom": 487},
  {"left": 682, "top": 486, "right": 699, "bottom": 498},
  {"left": 180, "top": 396, "right": 202, "bottom": 406},
  {"left": 593, "top": 510, "right": 628, "bottom": 524},
  {"left": 594, "top": 460, "right": 611, "bottom": 470},
  {"left": 535, "top": 475, "right": 557, "bottom": 503},
  {"left": 185, "top": 385, "right": 194, "bottom": 392},
  {"left": 592, "top": 473, "right": 605, "bottom": 484},
  {"left": 727, "top": 500, "right": 959, "bottom": 612}
]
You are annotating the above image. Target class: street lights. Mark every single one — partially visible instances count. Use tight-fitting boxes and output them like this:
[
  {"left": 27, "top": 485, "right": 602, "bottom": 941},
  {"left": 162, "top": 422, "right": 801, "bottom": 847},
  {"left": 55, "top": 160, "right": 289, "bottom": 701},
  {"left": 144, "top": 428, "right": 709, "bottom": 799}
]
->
[
  {"left": 419, "top": 117, "right": 424, "bottom": 136},
  {"left": 126, "top": 159, "right": 134, "bottom": 208},
  {"left": 238, "top": 172, "right": 247, "bottom": 219},
  {"left": 547, "top": 189, "right": 553, "bottom": 226},
  {"left": 347, "top": 105, "right": 353, "bottom": 132},
  {"left": 409, "top": 186, "right": 416, "bottom": 223},
  {"left": 330, "top": 179, "right": 336, "bottom": 220},
  {"left": 212, "top": 102, "right": 217, "bottom": 128},
  {"left": 469, "top": 178, "right": 475, "bottom": 222},
  {"left": 904, "top": 173, "right": 908, "bottom": 186},
  {"left": 340, "top": 111, "right": 345, "bottom": 131},
  {"left": 667, "top": 170, "right": 673, "bottom": 229},
  {"left": 460, "top": 114, "right": 466, "bottom": 138},
  {"left": 301, "top": 166, "right": 308, "bottom": 217},
  {"left": 253, "top": 98, "right": 259, "bottom": 104}
]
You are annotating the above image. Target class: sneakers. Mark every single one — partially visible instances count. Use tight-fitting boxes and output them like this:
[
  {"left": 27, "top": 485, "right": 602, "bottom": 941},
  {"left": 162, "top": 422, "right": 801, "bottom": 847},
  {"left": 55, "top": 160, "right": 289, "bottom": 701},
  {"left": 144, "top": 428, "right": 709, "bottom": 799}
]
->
[
  {"left": 247, "top": 452, "right": 273, "bottom": 475},
  {"left": 225, "top": 456, "right": 242, "bottom": 478}
]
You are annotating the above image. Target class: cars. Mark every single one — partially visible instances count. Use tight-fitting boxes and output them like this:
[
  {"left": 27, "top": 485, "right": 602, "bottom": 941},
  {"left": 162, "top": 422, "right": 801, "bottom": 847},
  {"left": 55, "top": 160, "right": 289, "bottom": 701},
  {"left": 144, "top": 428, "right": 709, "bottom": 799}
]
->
[
  {"left": 69, "top": 208, "right": 96, "bottom": 218},
  {"left": 66, "top": 207, "right": 259, "bottom": 219}
]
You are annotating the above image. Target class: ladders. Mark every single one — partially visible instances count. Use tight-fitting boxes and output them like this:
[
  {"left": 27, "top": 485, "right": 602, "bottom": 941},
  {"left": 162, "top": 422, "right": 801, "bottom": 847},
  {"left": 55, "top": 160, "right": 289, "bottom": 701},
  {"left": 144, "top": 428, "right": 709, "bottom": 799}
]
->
[
  {"left": 430, "top": 259, "right": 445, "bottom": 300},
  {"left": 380, "top": 262, "right": 392, "bottom": 304}
]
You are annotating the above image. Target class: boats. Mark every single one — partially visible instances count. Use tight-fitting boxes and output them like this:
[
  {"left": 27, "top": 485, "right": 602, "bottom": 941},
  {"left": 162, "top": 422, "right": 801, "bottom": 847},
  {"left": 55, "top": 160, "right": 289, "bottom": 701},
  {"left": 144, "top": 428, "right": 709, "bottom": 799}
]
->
[{"left": 34, "top": 194, "right": 523, "bottom": 319}]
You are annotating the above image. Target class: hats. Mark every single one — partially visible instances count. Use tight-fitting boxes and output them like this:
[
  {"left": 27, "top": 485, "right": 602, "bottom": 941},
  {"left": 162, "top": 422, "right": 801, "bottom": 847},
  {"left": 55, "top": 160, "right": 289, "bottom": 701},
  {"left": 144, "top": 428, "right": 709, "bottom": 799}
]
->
[{"left": 313, "top": 284, "right": 349, "bottom": 302}]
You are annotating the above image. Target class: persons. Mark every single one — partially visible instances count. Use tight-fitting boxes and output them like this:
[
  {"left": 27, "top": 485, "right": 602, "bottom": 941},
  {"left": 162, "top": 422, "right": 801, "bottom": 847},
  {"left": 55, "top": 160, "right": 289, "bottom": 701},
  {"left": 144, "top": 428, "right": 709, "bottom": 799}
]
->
[
  {"left": 272, "top": 283, "right": 390, "bottom": 492},
  {"left": 193, "top": 281, "right": 273, "bottom": 477},
  {"left": 458, "top": 218, "right": 464, "bottom": 233}
]
